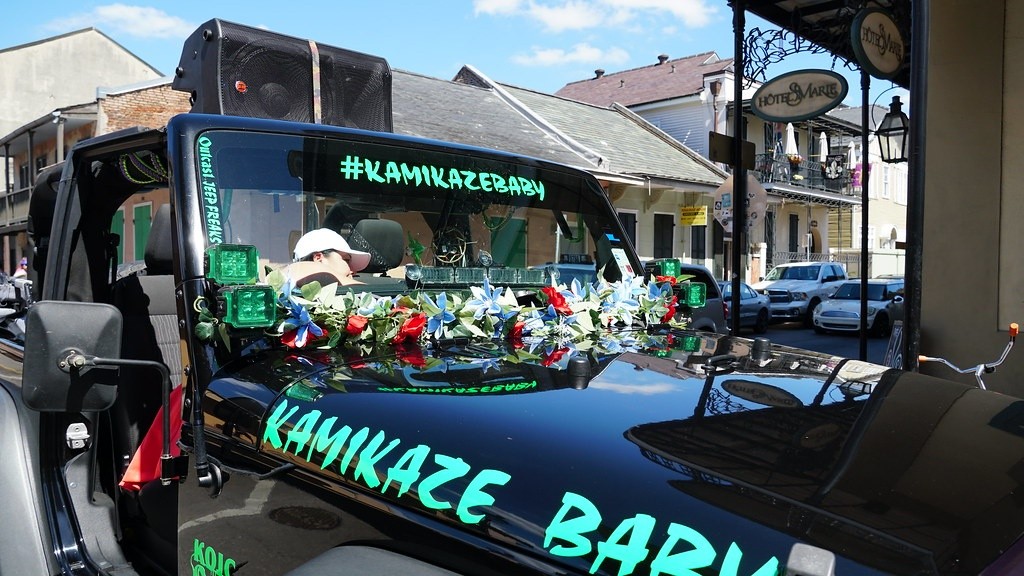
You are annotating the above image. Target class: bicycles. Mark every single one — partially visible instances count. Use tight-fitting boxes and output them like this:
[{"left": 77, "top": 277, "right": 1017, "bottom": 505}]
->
[{"left": 918, "top": 323, "right": 1019, "bottom": 391}]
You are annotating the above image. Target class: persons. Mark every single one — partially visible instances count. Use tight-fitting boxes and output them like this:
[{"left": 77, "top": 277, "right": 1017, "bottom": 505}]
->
[
  {"left": 266, "top": 228, "right": 371, "bottom": 288},
  {"left": 14, "top": 257, "right": 28, "bottom": 280}
]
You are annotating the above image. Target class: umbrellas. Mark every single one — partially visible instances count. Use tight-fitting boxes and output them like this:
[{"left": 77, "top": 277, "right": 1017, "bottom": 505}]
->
[
  {"left": 848, "top": 141, "right": 856, "bottom": 170},
  {"left": 786, "top": 123, "right": 798, "bottom": 155},
  {"left": 819, "top": 132, "right": 829, "bottom": 163}
]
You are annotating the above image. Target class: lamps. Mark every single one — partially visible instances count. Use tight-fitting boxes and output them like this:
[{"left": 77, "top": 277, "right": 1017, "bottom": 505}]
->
[{"left": 871, "top": 83, "right": 918, "bottom": 168}]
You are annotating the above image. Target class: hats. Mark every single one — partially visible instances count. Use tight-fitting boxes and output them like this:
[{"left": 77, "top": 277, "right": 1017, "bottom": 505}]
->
[{"left": 291, "top": 228, "right": 372, "bottom": 273}]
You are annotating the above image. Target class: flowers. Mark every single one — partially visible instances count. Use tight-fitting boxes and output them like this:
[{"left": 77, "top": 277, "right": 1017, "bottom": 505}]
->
[{"left": 196, "top": 276, "right": 697, "bottom": 354}]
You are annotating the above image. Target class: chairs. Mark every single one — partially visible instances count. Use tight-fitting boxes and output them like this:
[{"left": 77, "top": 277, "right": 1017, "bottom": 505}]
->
[
  {"left": 110, "top": 203, "right": 189, "bottom": 410},
  {"left": 345, "top": 217, "right": 406, "bottom": 278}
]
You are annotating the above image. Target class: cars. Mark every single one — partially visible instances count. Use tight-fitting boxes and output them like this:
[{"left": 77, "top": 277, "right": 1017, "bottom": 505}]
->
[
  {"left": 0, "top": 19, "right": 1024, "bottom": 575},
  {"left": 716, "top": 280, "right": 772, "bottom": 333},
  {"left": 813, "top": 277, "right": 906, "bottom": 337},
  {"left": 528, "top": 260, "right": 731, "bottom": 336},
  {"left": 744, "top": 262, "right": 848, "bottom": 325}
]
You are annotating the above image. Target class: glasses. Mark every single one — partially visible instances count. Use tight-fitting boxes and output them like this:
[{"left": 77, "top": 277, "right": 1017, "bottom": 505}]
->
[{"left": 20, "top": 261, "right": 28, "bottom": 266}]
